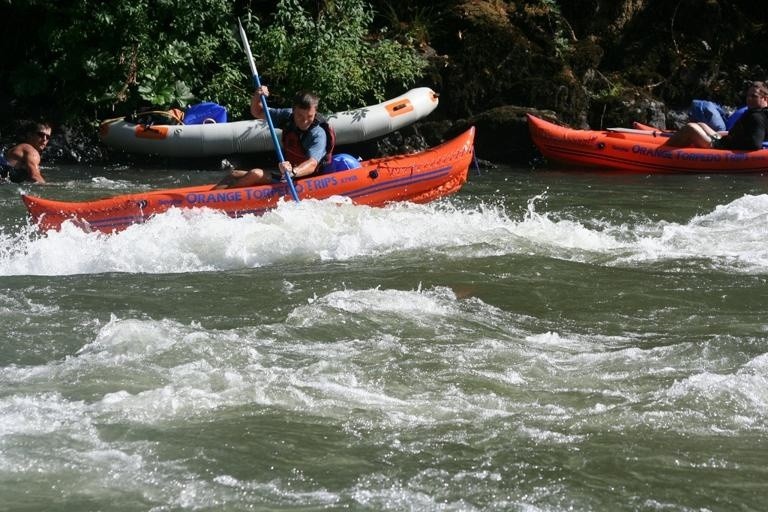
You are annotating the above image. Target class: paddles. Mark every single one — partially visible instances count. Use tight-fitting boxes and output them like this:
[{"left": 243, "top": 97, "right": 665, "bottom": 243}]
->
[
  {"left": 237, "top": 17, "right": 300, "bottom": 203},
  {"left": 607, "top": 127, "right": 674, "bottom": 138}
]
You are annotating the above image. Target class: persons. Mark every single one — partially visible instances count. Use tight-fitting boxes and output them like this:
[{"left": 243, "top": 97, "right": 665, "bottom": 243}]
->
[
  {"left": 207, "top": 84, "right": 335, "bottom": 192},
  {"left": 0, "top": 119, "right": 54, "bottom": 185},
  {"left": 657, "top": 78, "right": 768, "bottom": 151}
]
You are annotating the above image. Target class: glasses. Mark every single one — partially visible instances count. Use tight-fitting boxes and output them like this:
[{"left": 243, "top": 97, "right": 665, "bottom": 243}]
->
[{"left": 37, "top": 131, "right": 51, "bottom": 140}]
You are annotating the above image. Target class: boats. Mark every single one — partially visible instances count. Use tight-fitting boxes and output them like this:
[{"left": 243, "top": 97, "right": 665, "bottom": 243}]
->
[
  {"left": 15, "top": 125, "right": 478, "bottom": 241},
  {"left": 99, "top": 84, "right": 439, "bottom": 171},
  {"left": 524, "top": 108, "right": 767, "bottom": 171}
]
